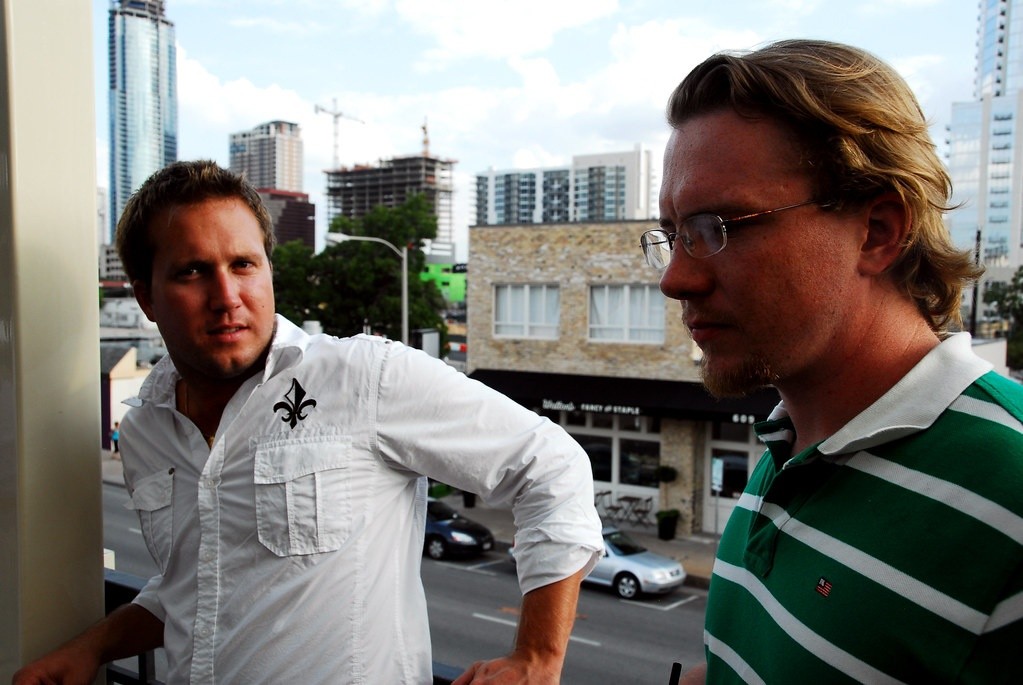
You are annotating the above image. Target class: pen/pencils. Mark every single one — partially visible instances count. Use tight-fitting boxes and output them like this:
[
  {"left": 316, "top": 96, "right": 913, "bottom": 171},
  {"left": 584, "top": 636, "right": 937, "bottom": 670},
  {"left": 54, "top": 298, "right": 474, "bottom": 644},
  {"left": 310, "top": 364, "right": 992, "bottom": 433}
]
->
[{"left": 669, "top": 661, "right": 683, "bottom": 685}]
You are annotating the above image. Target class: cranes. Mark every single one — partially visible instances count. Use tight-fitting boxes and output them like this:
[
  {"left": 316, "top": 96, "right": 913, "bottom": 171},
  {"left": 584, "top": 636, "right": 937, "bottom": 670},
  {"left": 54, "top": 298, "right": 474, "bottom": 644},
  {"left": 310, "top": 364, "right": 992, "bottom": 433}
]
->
[{"left": 314, "top": 96, "right": 365, "bottom": 172}]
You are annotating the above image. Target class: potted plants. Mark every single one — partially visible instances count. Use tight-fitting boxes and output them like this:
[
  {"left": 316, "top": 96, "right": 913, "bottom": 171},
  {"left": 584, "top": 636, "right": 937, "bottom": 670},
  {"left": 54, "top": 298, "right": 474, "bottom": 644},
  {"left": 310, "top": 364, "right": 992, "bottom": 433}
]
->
[{"left": 656, "top": 508, "right": 679, "bottom": 540}]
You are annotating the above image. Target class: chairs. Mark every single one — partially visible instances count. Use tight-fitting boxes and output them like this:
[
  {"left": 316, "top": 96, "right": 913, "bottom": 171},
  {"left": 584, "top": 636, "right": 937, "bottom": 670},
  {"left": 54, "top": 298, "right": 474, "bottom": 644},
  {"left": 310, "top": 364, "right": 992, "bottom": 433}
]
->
[{"left": 595, "top": 491, "right": 655, "bottom": 528}]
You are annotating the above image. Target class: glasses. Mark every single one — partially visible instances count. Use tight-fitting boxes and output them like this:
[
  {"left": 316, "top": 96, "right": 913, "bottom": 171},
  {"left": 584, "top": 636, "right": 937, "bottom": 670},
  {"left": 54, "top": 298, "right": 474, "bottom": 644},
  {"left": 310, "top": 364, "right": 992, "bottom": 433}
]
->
[{"left": 640, "top": 192, "right": 849, "bottom": 272}]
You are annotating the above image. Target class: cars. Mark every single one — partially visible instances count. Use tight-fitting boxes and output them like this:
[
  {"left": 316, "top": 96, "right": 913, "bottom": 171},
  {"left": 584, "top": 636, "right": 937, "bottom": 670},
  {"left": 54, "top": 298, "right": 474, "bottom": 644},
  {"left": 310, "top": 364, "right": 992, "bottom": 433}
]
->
[
  {"left": 424, "top": 495, "right": 497, "bottom": 561},
  {"left": 578, "top": 524, "right": 689, "bottom": 600}
]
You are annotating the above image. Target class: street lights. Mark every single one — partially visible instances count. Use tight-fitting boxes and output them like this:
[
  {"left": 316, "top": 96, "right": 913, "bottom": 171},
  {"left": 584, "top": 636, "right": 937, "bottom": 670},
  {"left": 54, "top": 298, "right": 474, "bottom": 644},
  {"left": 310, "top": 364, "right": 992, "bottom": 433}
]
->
[{"left": 323, "top": 230, "right": 409, "bottom": 346}]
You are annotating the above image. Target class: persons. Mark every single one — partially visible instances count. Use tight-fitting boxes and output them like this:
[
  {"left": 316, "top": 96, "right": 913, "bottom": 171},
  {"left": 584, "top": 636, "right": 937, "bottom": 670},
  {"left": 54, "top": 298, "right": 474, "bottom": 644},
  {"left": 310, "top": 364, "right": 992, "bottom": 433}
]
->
[
  {"left": 111, "top": 422, "right": 120, "bottom": 459},
  {"left": 658, "top": 38, "right": 1023, "bottom": 685},
  {"left": 10, "top": 160, "right": 605, "bottom": 685}
]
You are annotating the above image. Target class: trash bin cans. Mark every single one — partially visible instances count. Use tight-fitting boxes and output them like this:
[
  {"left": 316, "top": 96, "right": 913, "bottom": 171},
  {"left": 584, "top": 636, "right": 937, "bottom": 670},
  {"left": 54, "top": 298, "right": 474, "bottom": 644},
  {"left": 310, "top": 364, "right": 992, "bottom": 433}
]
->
[{"left": 654, "top": 509, "right": 678, "bottom": 542}]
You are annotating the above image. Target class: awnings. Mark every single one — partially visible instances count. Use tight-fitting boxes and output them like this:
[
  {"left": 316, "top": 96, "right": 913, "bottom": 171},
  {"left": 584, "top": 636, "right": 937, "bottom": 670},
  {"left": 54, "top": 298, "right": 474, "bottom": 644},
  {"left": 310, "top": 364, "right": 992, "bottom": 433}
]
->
[{"left": 466, "top": 368, "right": 782, "bottom": 426}]
